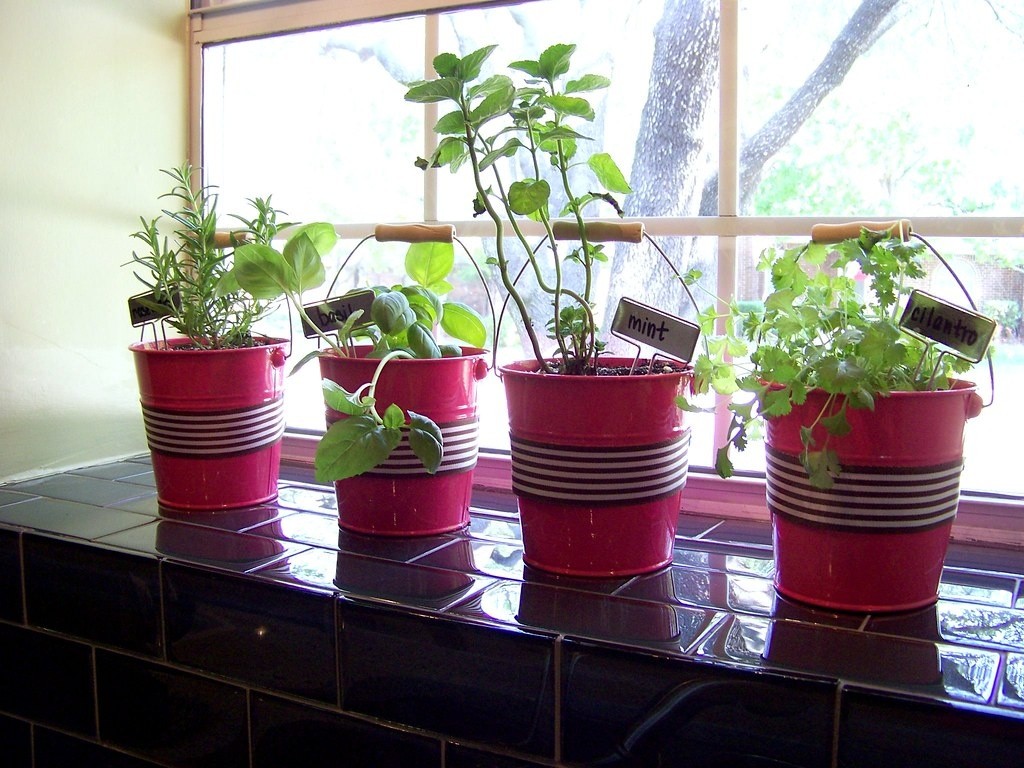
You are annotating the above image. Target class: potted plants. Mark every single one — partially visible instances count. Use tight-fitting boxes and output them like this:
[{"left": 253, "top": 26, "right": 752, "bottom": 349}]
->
[
  {"left": 677, "top": 219, "right": 997, "bottom": 619},
  {"left": 126, "top": 156, "right": 303, "bottom": 513},
  {"left": 403, "top": 42, "right": 710, "bottom": 573},
  {"left": 233, "top": 220, "right": 495, "bottom": 539}
]
